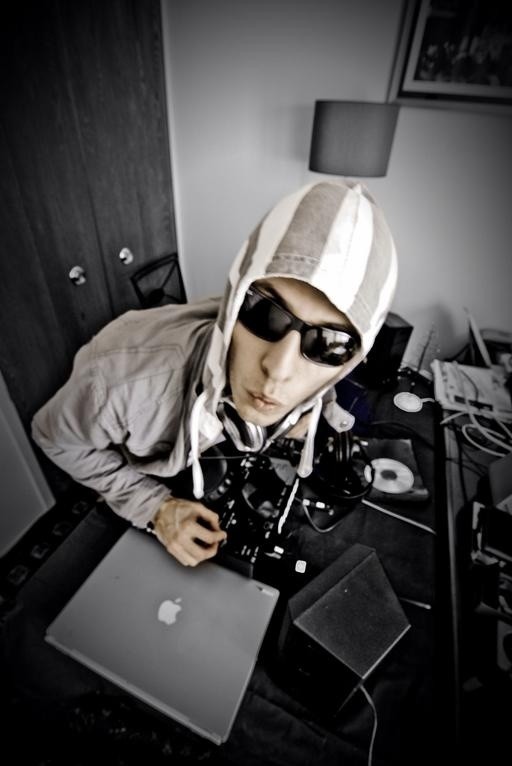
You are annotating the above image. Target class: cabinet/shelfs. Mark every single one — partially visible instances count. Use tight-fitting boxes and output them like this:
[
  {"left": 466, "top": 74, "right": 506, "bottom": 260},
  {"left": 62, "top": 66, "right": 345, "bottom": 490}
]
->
[{"left": 0, "top": 1, "right": 183, "bottom": 500}]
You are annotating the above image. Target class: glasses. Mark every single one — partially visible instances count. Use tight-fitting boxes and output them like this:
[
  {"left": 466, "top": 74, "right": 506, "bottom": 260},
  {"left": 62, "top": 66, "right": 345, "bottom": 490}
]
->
[{"left": 238, "top": 284, "right": 359, "bottom": 367}]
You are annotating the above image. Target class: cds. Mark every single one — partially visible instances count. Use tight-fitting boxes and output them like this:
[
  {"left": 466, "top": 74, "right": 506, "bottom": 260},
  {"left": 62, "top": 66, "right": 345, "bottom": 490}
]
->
[{"left": 363, "top": 457, "right": 415, "bottom": 494}]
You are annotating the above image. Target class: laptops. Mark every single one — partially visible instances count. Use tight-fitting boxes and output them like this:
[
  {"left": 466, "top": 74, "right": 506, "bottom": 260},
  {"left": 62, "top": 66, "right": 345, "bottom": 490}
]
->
[{"left": 44, "top": 527, "right": 281, "bottom": 747}]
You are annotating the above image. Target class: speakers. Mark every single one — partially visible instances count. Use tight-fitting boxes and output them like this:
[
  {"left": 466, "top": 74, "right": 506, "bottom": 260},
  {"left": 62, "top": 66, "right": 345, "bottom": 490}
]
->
[{"left": 267, "top": 542, "right": 413, "bottom": 720}]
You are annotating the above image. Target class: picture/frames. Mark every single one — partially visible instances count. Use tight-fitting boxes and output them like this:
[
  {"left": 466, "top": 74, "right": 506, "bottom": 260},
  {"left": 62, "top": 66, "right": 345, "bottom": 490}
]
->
[{"left": 386, "top": 0, "right": 511, "bottom": 114}]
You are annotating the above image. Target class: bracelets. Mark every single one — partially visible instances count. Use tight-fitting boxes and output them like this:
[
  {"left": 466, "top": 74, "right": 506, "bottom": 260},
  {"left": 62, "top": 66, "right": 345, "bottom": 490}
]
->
[{"left": 145, "top": 494, "right": 172, "bottom": 533}]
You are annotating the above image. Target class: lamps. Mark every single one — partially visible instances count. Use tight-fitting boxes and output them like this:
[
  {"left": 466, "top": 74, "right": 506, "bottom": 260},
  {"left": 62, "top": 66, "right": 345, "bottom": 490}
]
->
[{"left": 309, "top": 99, "right": 397, "bottom": 179}]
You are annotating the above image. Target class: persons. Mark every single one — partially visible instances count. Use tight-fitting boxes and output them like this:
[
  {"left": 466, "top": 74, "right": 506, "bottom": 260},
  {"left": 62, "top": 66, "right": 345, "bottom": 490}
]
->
[{"left": 31, "top": 176, "right": 401, "bottom": 569}]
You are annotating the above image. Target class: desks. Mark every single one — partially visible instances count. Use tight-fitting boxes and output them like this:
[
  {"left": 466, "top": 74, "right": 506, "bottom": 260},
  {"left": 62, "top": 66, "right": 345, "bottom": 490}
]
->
[{"left": 0, "top": 370, "right": 511, "bottom": 764}]
